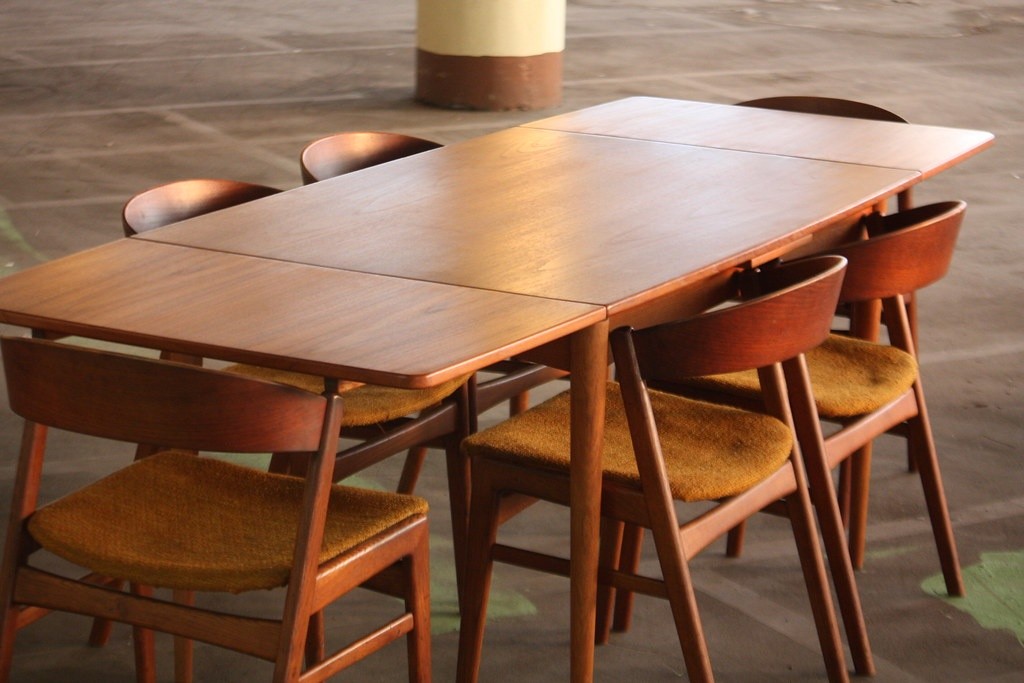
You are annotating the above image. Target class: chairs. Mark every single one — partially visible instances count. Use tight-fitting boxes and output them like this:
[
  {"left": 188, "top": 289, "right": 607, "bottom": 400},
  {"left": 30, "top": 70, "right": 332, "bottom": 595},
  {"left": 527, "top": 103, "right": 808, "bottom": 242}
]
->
[{"left": 0, "top": 96, "right": 966, "bottom": 683}]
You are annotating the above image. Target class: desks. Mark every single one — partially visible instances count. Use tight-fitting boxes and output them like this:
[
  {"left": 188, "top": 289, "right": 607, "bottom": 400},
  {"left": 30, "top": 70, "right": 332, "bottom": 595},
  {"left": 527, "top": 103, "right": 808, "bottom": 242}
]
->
[{"left": 0, "top": 96, "right": 995, "bottom": 683}]
